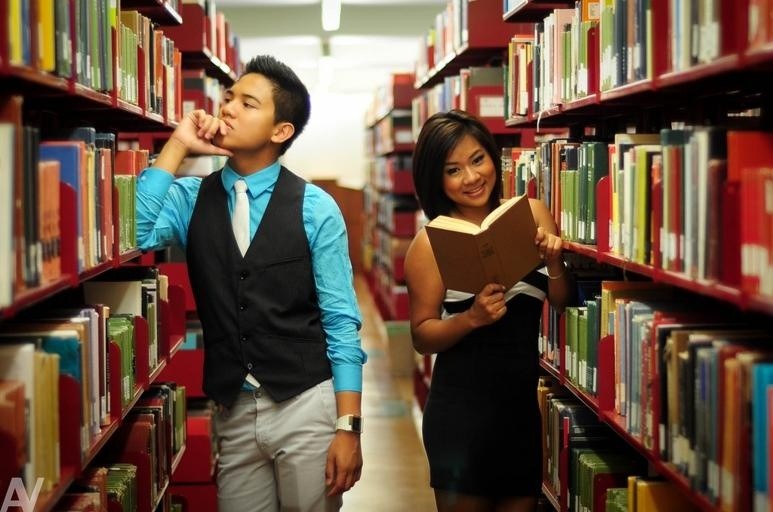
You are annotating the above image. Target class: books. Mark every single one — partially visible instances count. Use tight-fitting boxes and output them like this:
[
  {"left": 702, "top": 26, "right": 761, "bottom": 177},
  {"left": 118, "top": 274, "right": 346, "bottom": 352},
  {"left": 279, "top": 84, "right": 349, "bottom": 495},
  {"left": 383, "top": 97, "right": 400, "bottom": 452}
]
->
[{"left": 1, "top": 1, "right": 773, "bottom": 511}]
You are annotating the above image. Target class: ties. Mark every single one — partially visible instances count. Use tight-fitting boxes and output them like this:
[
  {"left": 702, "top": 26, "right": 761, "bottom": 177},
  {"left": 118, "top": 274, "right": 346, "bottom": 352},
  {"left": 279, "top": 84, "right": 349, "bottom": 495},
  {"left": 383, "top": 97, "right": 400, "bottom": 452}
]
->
[{"left": 232, "top": 179, "right": 251, "bottom": 258}]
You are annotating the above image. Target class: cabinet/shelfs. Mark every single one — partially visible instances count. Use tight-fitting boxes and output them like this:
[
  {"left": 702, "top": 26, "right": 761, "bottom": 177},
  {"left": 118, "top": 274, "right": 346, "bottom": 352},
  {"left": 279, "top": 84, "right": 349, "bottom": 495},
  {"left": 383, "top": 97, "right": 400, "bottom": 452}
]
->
[
  {"left": 362, "top": 0, "right": 772, "bottom": 511},
  {"left": 0, "top": 0, "right": 247, "bottom": 512}
]
